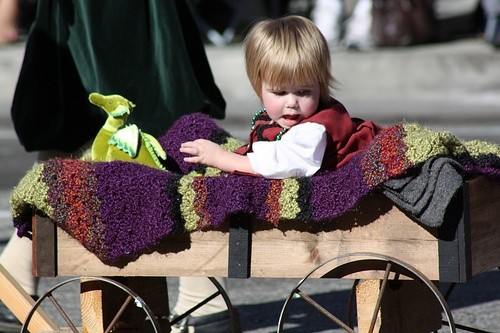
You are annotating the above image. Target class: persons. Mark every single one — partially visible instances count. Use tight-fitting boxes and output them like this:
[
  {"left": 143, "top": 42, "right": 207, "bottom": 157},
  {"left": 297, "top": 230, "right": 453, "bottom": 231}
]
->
[
  {"left": 179, "top": 15, "right": 358, "bottom": 180},
  {"left": 0, "top": 0, "right": 227, "bottom": 165}
]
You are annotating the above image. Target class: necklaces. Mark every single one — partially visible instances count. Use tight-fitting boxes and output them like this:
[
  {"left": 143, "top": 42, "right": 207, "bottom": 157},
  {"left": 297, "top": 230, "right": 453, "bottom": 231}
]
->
[{"left": 250, "top": 107, "right": 286, "bottom": 142}]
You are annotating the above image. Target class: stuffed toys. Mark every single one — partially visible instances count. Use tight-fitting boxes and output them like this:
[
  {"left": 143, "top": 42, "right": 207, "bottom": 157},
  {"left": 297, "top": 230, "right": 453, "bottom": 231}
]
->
[{"left": 87, "top": 91, "right": 167, "bottom": 170}]
